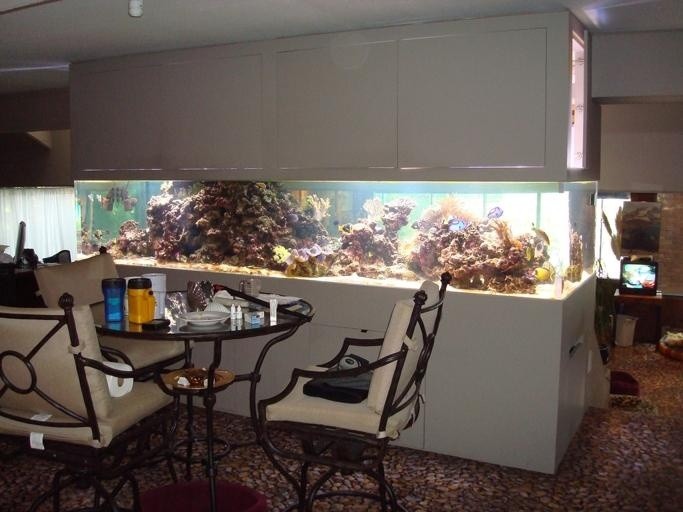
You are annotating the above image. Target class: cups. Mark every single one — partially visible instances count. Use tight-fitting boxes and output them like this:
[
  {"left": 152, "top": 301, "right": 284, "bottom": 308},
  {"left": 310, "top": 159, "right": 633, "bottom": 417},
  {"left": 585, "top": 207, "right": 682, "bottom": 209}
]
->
[
  {"left": 141, "top": 272, "right": 169, "bottom": 321},
  {"left": 128, "top": 277, "right": 155, "bottom": 324},
  {"left": 101, "top": 277, "right": 127, "bottom": 324}
]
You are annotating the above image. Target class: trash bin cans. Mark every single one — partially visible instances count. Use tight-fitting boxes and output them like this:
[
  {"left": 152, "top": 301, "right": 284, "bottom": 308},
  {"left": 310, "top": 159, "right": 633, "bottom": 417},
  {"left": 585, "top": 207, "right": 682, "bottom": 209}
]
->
[{"left": 614, "top": 314, "right": 638, "bottom": 347}]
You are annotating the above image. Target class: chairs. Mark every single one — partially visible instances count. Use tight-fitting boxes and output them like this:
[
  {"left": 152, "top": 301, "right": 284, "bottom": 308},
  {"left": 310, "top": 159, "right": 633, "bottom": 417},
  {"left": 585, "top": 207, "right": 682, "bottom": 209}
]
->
[
  {"left": 255, "top": 273, "right": 451, "bottom": 511},
  {"left": 0, "top": 292, "right": 192, "bottom": 511},
  {"left": 33, "top": 246, "right": 195, "bottom": 485}
]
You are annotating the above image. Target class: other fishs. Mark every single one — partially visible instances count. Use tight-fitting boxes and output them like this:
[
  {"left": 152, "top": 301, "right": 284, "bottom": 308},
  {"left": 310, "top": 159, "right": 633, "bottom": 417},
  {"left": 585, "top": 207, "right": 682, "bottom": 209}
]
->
[
  {"left": 531, "top": 266, "right": 555, "bottom": 283},
  {"left": 487, "top": 207, "right": 503, "bottom": 220},
  {"left": 447, "top": 218, "right": 465, "bottom": 231},
  {"left": 338, "top": 222, "right": 353, "bottom": 236},
  {"left": 525, "top": 244, "right": 535, "bottom": 267},
  {"left": 366, "top": 251, "right": 376, "bottom": 257},
  {"left": 403, "top": 242, "right": 416, "bottom": 249},
  {"left": 94, "top": 229, "right": 103, "bottom": 240},
  {"left": 288, "top": 214, "right": 299, "bottom": 223},
  {"left": 533, "top": 227, "right": 549, "bottom": 246}
]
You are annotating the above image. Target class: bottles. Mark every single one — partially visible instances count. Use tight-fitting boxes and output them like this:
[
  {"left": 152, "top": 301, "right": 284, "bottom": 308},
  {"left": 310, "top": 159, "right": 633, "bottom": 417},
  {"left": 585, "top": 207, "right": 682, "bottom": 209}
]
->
[
  {"left": 230, "top": 319, "right": 244, "bottom": 332},
  {"left": 231, "top": 303, "right": 242, "bottom": 320}
]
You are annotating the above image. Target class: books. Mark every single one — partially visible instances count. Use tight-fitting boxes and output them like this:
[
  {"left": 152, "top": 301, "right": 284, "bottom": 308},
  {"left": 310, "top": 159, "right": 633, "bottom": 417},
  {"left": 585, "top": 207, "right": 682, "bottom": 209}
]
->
[{"left": 214, "top": 296, "right": 250, "bottom": 308}]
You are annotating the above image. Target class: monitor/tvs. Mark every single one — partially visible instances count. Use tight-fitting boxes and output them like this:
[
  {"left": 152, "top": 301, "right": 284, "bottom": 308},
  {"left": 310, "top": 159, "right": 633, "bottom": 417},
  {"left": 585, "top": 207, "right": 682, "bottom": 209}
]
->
[{"left": 619, "top": 261, "right": 658, "bottom": 295}]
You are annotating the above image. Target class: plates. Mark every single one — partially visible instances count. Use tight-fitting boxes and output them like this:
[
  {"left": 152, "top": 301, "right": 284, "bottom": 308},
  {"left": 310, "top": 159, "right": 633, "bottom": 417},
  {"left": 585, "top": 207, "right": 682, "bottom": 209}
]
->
[
  {"left": 178, "top": 324, "right": 230, "bottom": 333},
  {"left": 177, "top": 310, "right": 231, "bottom": 324}
]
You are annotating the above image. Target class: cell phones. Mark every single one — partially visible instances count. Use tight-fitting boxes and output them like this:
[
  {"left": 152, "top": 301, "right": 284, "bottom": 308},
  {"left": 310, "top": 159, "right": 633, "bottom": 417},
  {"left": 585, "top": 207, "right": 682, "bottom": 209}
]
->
[{"left": 142, "top": 319, "right": 171, "bottom": 330}]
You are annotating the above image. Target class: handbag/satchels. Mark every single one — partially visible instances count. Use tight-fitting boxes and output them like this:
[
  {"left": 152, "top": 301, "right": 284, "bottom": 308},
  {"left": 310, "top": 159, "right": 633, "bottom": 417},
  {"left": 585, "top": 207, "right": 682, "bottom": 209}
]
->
[
  {"left": 304, "top": 353, "right": 374, "bottom": 404},
  {"left": 186, "top": 279, "right": 214, "bottom": 310}
]
